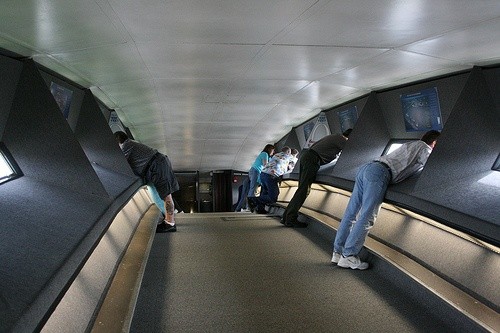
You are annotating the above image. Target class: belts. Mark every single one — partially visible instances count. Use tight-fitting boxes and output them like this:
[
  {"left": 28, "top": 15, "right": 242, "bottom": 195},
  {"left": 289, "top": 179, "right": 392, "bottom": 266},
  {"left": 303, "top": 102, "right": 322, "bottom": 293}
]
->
[
  {"left": 145, "top": 152, "right": 161, "bottom": 176},
  {"left": 374, "top": 161, "right": 392, "bottom": 185}
]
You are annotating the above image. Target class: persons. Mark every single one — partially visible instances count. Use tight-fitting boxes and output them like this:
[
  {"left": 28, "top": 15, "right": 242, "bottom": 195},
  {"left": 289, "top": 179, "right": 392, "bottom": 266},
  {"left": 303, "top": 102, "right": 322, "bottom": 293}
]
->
[
  {"left": 113, "top": 130, "right": 181, "bottom": 233},
  {"left": 234, "top": 174, "right": 251, "bottom": 212},
  {"left": 245, "top": 143, "right": 276, "bottom": 212},
  {"left": 330, "top": 128, "right": 443, "bottom": 270},
  {"left": 247, "top": 144, "right": 299, "bottom": 213},
  {"left": 281, "top": 126, "right": 354, "bottom": 228},
  {"left": 172, "top": 193, "right": 186, "bottom": 214},
  {"left": 282, "top": 147, "right": 299, "bottom": 174}
]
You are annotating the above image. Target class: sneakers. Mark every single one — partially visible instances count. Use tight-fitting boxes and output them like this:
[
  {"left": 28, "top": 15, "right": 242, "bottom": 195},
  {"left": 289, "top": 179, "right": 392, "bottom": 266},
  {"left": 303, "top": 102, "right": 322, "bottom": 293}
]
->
[
  {"left": 336, "top": 255, "right": 369, "bottom": 270},
  {"left": 330, "top": 252, "right": 342, "bottom": 263}
]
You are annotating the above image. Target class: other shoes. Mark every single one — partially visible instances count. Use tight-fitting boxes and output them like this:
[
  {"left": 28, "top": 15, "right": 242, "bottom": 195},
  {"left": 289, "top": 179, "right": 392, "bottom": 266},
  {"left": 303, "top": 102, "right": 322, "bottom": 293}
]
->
[
  {"left": 157, "top": 221, "right": 175, "bottom": 226},
  {"left": 248, "top": 196, "right": 256, "bottom": 213},
  {"left": 256, "top": 207, "right": 269, "bottom": 214},
  {"left": 280, "top": 217, "right": 287, "bottom": 224},
  {"left": 285, "top": 220, "right": 307, "bottom": 227},
  {"left": 156, "top": 225, "right": 176, "bottom": 232}
]
